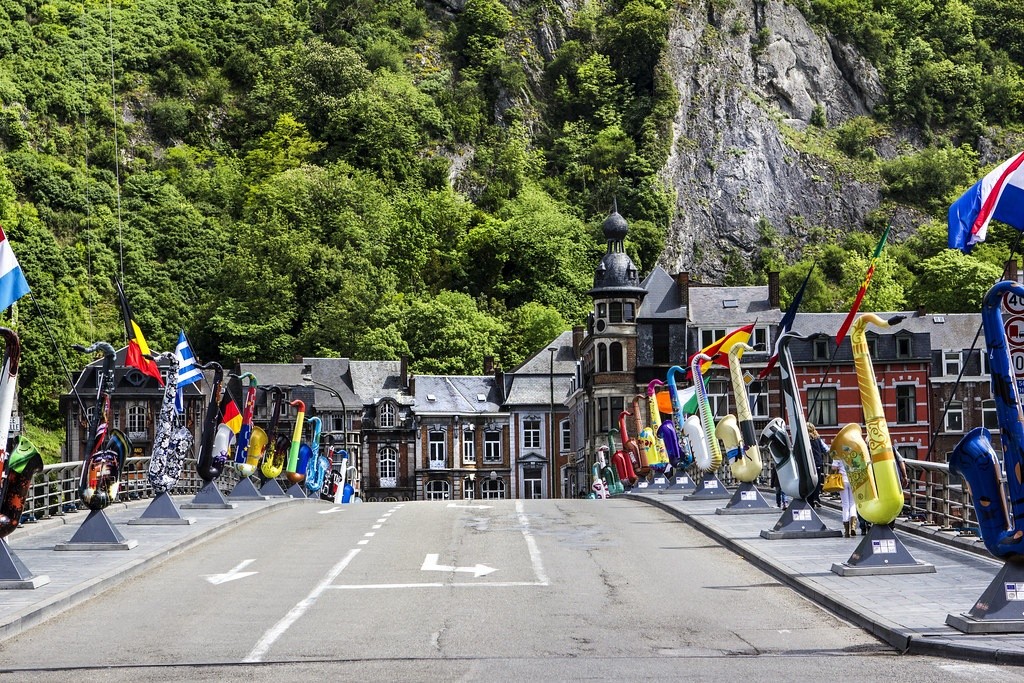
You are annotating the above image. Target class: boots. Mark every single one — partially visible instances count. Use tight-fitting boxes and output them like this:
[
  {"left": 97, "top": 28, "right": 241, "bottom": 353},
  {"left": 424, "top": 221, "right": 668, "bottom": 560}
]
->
[
  {"left": 851, "top": 517, "right": 856, "bottom": 536},
  {"left": 843, "top": 521, "right": 850, "bottom": 538}
]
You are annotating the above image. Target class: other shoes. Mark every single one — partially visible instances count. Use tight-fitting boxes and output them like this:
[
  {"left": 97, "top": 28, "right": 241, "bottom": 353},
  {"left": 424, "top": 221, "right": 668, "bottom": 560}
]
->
[
  {"left": 782, "top": 504, "right": 787, "bottom": 510},
  {"left": 814, "top": 498, "right": 822, "bottom": 507},
  {"left": 861, "top": 529, "right": 866, "bottom": 535}
]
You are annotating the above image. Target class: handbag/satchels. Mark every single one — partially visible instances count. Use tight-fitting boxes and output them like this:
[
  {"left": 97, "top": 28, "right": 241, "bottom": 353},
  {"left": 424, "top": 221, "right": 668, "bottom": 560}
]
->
[{"left": 822, "top": 467, "right": 845, "bottom": 492}]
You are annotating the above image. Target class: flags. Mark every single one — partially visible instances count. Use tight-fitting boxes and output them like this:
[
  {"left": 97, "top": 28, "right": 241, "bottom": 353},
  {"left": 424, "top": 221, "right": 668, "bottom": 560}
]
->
[
  {"left": 114, "top": 273, "right": 166, "bottom": 388},
  {"left": 834, "top": 217, "right": 894, "bottom": 348},
  {"left": 946, "top": 151, "right": 1023, "bottom": 253},
  {"left": 0, "top": 223, "right": 30, "bottom": 313},
  {"left": 214, "top": 361, "right": 244, "bottom": 436},
  {"left": 684, "top": 323, "right": 755, "bottom": 379},
  {"left": 656, "top": 376, "right": 709, "bottom": 415},
  {"left": 759, "top": 265, "right": 815, "bottom": 379},
  {"left": 174, "top": 328, "right": 205, "bottom": 412}
]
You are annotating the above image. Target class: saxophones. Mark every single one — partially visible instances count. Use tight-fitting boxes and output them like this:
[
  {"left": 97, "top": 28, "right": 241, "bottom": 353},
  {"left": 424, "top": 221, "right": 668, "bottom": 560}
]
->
[
  {"left": 0, "top": 323, "right": 44, "bottom": 538},
  {"left": 303, "top": 414, "right": 363, "bottom": 503},
  {"left": 140, "top": 351, "right": 194, "bottom": 494},
  {"left": 257, "top": 383, "right": 292, "bottom": 478},
  {"left": 657, "top": 362, "right": 691, "bottom": 469},
  {"left": 272, "top": 398, "right": 314, "bottom": 483},
  {"left": 714, "top": 341, "right": 767, "bottom": 482},
  {"left": 947, "top": 281, "right": 1024, "bottom": 566},
  {"left": 70, "top": 340, "right": 136, "bottom": 511},
  {"left": 828, "top": 311, "right": 909, "bottom": 524},
  {"left": 190, "top": 361, "right": 236, "bottom": 482},
  {"left": 589, "top": 378, "right": 669, "bottom": 498},
  {"left": 228, "top": 370, "right": 269, "bottom": 478},
  {"left": 758, "top": 330, "right": 831, "bottom": 501},
  {"left": 681, "top": 353, "right": 723, "bottom": 474}
]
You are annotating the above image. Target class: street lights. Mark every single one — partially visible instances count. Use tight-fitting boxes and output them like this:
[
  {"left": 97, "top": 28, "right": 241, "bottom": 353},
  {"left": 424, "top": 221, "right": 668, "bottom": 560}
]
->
[
  {"left": 548, "top": 346, "right": 559, "bottom": 498},
  {"left": 297, "top": 378, "right": 347, "bottom": 485}
]
